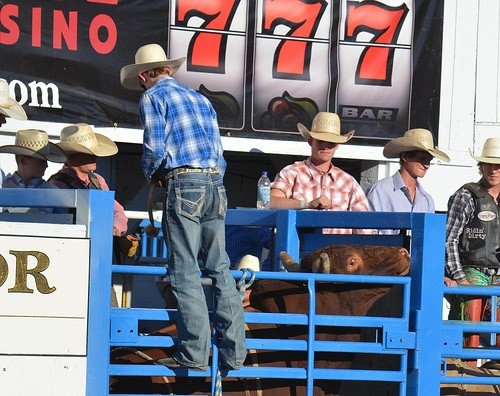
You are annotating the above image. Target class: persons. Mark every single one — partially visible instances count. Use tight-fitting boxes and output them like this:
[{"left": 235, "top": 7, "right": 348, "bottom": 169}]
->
[
  {"left": 270, "top": 113, "right": 378, "bottom": 235},
  {"left": 0, "top": 44, "right": 262, "bottom": 371},
  {"left": 366, "top": 128, "right": 459, "bottom": 320},
  {"left": 443, "top": 138, "right": 500, "bottom": 356}
]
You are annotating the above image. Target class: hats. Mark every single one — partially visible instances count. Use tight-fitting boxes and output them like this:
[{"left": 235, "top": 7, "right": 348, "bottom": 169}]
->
[
  {"left": 55, "top": 123, "right": 118, "bottom": 157},
  {"left": 473, "top": 138, "right": 500, "bottom": 165},
  {"left": 0, "top": 78, "right": 28, "bottom": 122},
  {"left": 383, "top": 128, "right": 451, "bottom": 163},
  {"left": 0, "top": 129, "right": 68, "bottom": 163},
  {"left": 119, "top": 43, "right": 187, "bottom": 91},
  {"left": 297, "top": 112, "right": 356, "bottom": 144}
]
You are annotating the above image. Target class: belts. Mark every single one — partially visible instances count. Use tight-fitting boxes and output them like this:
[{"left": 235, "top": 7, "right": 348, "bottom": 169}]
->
[{"left": 165, "top": 167, "right": 220, "bottom": 179}]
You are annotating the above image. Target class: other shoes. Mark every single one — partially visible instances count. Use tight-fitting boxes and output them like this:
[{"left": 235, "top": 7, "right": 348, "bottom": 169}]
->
[
  {"left": 153, "top": 357, "right": 208, "bottom": 371},
  {"left": 220, "top": 355, "right": 234, "bottom": 370}
]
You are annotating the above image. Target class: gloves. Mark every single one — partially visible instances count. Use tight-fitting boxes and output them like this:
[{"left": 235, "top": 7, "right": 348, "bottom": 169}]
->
[{"left": 115, "top": 233, "right": 141, "bottom": 258}]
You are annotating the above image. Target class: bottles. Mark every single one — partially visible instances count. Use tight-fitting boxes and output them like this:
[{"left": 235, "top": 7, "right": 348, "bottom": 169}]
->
[{"left": 257, "top": 171, "right": 271, "bottom": 210}]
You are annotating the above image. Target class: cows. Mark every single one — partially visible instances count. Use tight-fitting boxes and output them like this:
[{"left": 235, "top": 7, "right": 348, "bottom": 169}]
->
[{"left": 110, "top": 242, "right": 413, "bottom": 396}]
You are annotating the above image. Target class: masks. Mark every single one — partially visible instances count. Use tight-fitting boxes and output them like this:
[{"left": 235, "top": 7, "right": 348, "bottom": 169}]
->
[{"left": 138, "top": 73, "right": 147, "bottom": 90}]
